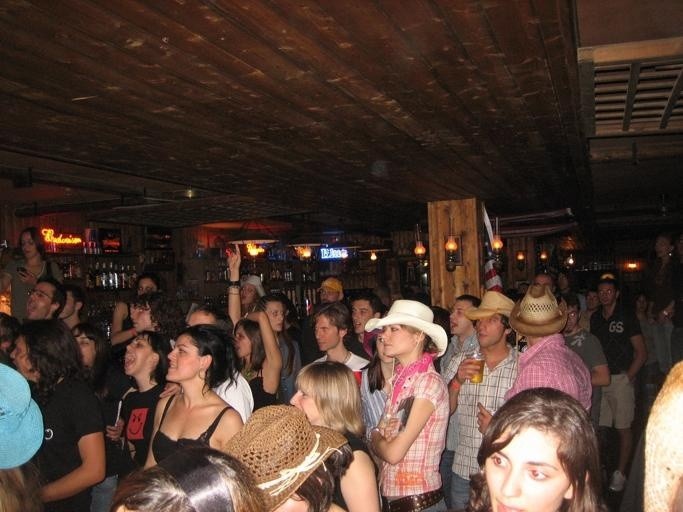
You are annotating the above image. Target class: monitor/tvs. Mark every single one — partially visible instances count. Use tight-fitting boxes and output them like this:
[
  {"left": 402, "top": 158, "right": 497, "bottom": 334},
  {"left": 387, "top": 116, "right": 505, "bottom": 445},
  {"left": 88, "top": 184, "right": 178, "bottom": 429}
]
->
[{"left": 320, "top": 248, "right": 348, "bottom": 260}]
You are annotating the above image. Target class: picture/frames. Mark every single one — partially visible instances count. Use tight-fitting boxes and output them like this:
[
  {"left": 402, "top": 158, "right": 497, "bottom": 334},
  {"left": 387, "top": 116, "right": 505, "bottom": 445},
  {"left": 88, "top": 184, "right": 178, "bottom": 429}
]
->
[{"left": 446, "top": 235, "right": 462, "bottom": 266}]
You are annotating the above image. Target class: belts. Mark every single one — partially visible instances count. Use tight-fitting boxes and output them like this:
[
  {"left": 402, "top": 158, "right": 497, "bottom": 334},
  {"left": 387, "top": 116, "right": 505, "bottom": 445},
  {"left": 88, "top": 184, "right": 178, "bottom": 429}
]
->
[{"left": 382, "top": 489, "right": 443, "bottom": 512}]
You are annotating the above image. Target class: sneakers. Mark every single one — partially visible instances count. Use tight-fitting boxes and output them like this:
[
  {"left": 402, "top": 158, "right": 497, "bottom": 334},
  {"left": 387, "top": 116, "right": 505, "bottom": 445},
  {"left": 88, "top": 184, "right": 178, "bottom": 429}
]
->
[{"left": 607, "top": 469, "right": 626, "bottom": 493}]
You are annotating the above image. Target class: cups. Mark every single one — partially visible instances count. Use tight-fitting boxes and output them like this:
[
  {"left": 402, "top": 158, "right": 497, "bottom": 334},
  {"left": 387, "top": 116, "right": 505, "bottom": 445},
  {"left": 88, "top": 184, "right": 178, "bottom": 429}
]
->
[{"left": 464, "top": 350, "right": 485, "bottom": 384}]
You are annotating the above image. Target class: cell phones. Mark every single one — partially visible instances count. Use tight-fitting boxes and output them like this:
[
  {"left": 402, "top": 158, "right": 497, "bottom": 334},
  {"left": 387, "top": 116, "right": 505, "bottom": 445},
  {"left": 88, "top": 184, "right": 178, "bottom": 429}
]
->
[{"left": 16, "top": 266, "right": 26, "bottom": 271}]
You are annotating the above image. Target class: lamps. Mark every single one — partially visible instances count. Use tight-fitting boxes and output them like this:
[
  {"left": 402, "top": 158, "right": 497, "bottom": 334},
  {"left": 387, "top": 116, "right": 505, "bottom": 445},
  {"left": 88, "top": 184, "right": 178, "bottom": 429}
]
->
[
  {"left": 490, "top": 233, "right": 507, "bottom": 271},
  {"left": 444, "top": 236, "right": 461, "bottom": 274},
  {"left": 515, "top": 250, "right": 525, "bottom": 272},
  {"left": 225, "top": 218, "right": 391, "bottom": 264},
  {"left": 413, "top": 240, "right": 431, "bottom": 273}
]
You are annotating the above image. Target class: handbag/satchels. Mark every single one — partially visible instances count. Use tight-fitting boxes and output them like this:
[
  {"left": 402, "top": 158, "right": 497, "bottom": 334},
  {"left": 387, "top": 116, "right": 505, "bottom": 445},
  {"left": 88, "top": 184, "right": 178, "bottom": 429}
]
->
[{"left": 88, "top": 387, "right": 134, "bottom": 512}]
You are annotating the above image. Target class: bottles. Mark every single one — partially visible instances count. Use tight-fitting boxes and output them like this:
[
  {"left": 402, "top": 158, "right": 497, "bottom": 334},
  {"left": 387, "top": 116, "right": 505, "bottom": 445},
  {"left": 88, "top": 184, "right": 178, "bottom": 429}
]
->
[
  {"left": 204, "top": 292, "right": 227, "bottom": 310},
  {"left": 83, "top": 260, "right": 138, "bottom": 289},
  {"left": 204, "top": 258, "right": 260, "bottom": 281},
  {"left": 269, "top": 261, "right": 380, "bottom": 316},
  {"left": 86, "top": 300, "right": 118, "bottom": 318},
  {"left": 61, "top": 255, "right": 73, "bottom": 280}
]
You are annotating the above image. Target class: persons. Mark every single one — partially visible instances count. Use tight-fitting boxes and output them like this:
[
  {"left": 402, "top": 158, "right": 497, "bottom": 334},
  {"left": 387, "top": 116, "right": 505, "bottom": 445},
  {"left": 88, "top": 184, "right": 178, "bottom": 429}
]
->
[{"left": 0, "top": 227, "right": 683, "bottom": 512}]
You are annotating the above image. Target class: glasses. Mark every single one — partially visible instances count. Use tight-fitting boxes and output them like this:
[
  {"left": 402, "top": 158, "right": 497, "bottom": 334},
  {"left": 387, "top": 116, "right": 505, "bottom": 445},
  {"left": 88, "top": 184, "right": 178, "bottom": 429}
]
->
[{"left": 27, "top": 288, "right": 52, "bottom": 300}]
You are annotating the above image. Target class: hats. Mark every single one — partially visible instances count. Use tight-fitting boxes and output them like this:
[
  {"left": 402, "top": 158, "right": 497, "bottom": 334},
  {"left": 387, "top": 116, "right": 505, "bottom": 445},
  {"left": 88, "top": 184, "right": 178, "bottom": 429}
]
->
[
  {"left": 223, "top": 403, "right": 350, "bottom": 512},
  {"left": 0, "top": 362, "right": 45, "bottom": 470},
  {"left": 316, "top": 277, "right": 344, "bottom": 293},
  {"left": 464, "top": 291, "right": 514, "bottom": 321},
  {"left": 508, "top": 283, "right": 567, "bottom": 338},
  {"left": 364, "top": 299, "right": 448, "bottom": 358}
]
